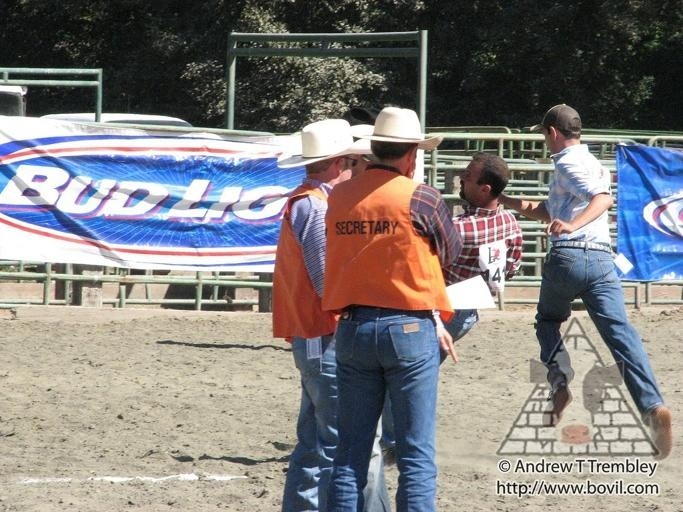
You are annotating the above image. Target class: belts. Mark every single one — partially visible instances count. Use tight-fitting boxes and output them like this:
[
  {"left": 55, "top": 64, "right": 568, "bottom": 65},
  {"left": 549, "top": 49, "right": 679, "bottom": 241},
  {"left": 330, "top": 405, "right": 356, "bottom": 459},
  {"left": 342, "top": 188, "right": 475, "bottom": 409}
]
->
[{"left": 553, "top": 240, "right": 611, "bottom": 253}]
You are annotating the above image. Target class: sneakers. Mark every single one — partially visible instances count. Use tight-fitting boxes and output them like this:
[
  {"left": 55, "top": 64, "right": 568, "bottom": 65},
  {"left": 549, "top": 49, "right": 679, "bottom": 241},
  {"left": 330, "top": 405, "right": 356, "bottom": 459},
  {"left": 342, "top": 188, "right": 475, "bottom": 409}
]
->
[
  {"left": 649, "top": 406, "right": 673, "bottom": 460},
  {"left": 543, "top": 383, "right": 571, "bottom": 426}
]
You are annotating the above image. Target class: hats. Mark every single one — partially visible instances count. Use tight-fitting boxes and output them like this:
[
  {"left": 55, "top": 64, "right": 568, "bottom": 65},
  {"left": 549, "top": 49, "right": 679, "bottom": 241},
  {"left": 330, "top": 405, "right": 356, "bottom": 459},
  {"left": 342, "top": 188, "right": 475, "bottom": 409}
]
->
[
  {"left": 530, "top": 104, "right": 583, "bottom": 132},
  {"left": 276, "top": 107, "right": 441, "bottom": 169}
]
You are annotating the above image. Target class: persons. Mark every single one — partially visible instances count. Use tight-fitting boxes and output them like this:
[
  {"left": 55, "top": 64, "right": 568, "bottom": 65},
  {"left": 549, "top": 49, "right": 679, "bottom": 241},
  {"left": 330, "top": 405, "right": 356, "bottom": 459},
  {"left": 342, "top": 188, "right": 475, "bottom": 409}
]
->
[
  {"left": 379, "top": 151, "right": 524, "bottom": 466},
  {"left": 271, "top": 106, "right": 462, "bottom": 512},
  {"left": 497, "top": 102, "right": 673, "bottom": 462}
]
